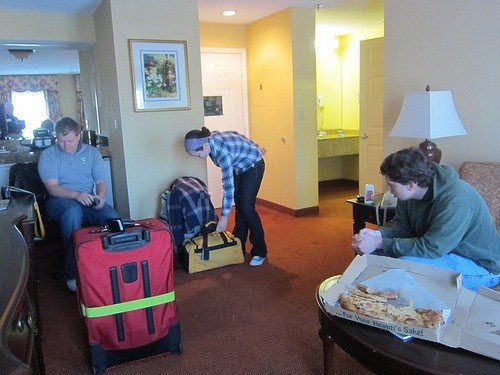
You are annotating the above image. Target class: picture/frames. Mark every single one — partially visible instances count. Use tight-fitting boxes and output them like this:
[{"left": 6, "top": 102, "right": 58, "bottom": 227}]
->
[{"left": 127, "top": 37, "right": 193, "bottom": 112}]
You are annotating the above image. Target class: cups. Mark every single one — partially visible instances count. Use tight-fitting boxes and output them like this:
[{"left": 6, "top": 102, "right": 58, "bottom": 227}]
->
[{"left": 338, "top": 131, "right": 343, "bottom": 137}]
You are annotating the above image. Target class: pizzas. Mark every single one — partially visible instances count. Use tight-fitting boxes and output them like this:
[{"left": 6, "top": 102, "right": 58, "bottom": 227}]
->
[{"left": 341, "top": 283, "right": 444, "bottom": 328}]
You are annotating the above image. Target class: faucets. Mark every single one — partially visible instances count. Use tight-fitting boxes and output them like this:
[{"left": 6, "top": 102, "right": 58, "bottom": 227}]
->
[{"left": 337, "top": 130, "right": 344, "bottom": 137}]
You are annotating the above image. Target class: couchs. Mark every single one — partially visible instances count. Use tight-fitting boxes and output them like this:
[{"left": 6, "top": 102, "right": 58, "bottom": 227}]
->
[{"left": 459, "top": 160, "right": 500, "bottom": 294}]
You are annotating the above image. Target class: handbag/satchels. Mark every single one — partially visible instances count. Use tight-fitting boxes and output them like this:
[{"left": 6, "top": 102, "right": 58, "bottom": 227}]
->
[
  {"left": 160, "top": 177, "right": 219, "bottom": 263},
  {"left": 184, "top": 221, "right": 245, "bottom": 274}
]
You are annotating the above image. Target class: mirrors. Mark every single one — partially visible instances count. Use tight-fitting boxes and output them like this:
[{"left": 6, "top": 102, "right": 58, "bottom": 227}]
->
[{"left": 0, "top": 39, "right": 101, "bottom": 141}]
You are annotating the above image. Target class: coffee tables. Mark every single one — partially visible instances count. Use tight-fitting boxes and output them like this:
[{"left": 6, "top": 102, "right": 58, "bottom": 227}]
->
[{"left": 319, "top": 306, "right": 500, "bottom": 375}]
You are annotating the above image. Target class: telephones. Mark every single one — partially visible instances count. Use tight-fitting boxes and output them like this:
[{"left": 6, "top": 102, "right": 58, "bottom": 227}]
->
[{"left": 381, "top": 191, "right": 397, "bottom": 209}]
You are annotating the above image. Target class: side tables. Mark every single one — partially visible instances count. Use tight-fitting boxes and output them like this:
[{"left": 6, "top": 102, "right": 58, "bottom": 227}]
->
[{"left": 344, "top": 194, "right": 398, "bottom": 259}]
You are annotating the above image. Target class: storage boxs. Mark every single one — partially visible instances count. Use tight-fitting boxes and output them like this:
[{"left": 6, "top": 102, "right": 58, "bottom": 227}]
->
[{"left": 320, "top": 252, "right": 500, "bottom": 361}]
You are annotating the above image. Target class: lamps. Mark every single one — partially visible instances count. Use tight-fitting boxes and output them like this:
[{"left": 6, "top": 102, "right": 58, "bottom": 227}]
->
[
  {"left": 388, "top": 82, "right": 470, "bottom": 163},
  {"left": 8, "top": 49, "right": 34, "bottom": 62}
]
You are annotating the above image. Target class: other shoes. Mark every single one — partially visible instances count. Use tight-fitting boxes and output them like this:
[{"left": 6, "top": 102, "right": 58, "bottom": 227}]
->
[
  {"left": 250, "top": 256, "right": 266, "bottom": 266},
  {"left": 66, "top": 278, "right": 78, "bottom": 291}
]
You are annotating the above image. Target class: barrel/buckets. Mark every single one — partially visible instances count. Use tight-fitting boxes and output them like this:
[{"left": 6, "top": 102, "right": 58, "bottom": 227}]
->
[{"left": 83, "top": 130, "right": 95, "bottom": 147}]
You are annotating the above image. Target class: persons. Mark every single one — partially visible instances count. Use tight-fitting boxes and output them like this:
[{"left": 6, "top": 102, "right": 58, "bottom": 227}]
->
[
  {"left": 184, "top": 127, "right": 267, "bottom": 266},
  {"left": 5, "top": 103, "right": 25, "bottom": 139},
  {"left": 38, "top": 117, "right": 119, "bottom": 291},
  {"left": 351, "top": 146, "right": 500, "bottom": 291}
]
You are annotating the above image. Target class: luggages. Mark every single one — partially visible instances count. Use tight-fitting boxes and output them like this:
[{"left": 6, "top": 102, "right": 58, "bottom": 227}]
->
[{"left": 72, "top": 217, "right": 183, "bottom": 375}]
